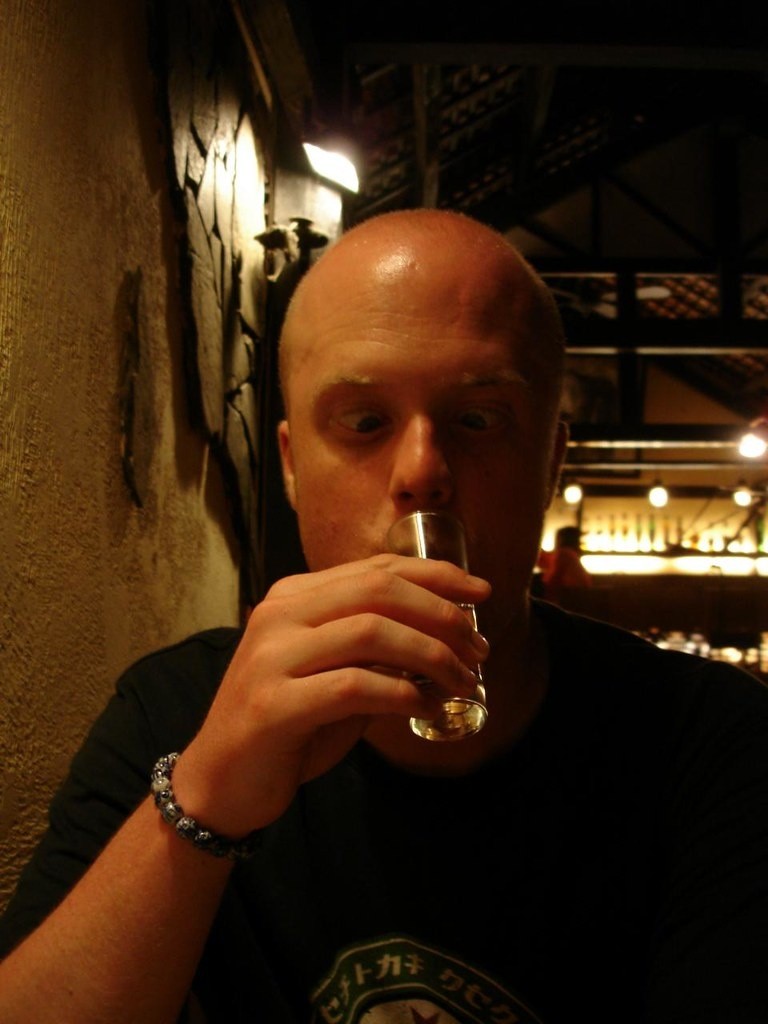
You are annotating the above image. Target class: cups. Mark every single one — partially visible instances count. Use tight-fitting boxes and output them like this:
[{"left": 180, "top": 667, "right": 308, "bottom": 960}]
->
[{"left": 385, "top": 509, "right": 488, "bottom": 744}]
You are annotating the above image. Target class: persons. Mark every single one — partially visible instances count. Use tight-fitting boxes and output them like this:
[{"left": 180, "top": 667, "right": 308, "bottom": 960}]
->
[
  {"left": 0, "top": 208, "right": 767, "bottom": 1024},
  {"left": 541, "top": 523, "right": 592, "bottom": 588}
]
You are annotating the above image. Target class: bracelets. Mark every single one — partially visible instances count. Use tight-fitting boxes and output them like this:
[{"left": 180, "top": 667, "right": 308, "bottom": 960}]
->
[{"left": 151, "top": 750, "right": 266, "bottom": 856}]
